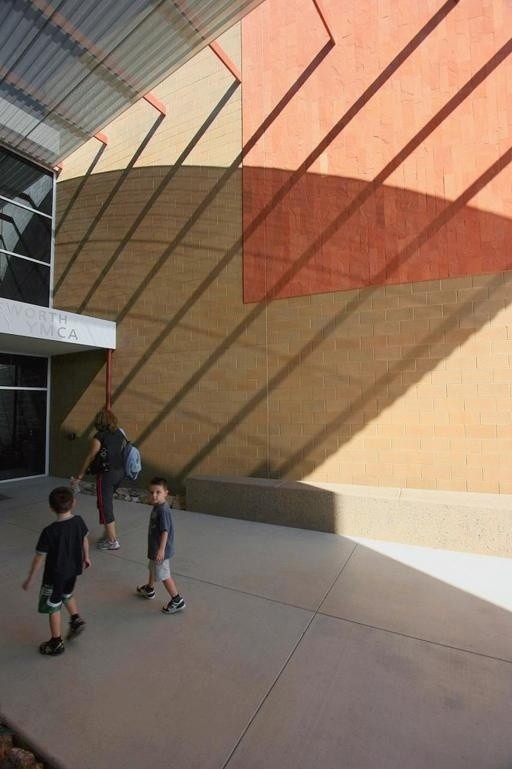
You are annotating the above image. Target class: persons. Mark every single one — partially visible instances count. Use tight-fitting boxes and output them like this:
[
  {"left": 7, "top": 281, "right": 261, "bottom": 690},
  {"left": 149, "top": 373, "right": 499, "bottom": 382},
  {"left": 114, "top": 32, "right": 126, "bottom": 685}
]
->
[
  {"left": 72, "top": 408, "right": 128, "bottom": 551},
  {"left": 137, "top": 477, "right": 186, "bottom": 614},
  {"left": 23, "top": 487, "right": 91, "bottom": 656}
]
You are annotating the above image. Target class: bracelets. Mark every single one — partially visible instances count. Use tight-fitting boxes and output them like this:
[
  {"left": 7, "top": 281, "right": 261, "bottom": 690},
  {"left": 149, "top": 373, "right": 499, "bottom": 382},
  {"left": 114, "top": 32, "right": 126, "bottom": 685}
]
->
[{"left": 76, "top": 476, "right": 83, "bottom": 481}]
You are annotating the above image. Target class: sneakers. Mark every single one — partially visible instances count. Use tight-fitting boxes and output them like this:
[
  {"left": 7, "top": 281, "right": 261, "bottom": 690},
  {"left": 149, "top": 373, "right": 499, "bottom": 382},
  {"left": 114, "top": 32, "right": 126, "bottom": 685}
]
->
[
  {"left": 138, "top": 585, "right": 155, "bottom": 600},
  {"left": 40, "top": 640, "right": 65, "bottom": 655},
  {"left": 96, "top": 536, "right": 120, "bottom": 549},
  {"left": 66, "top": 617, "right": 86, "bottom": 641},
  {"left": 162, "top": 599, "right": 185, "bottom": 614}
]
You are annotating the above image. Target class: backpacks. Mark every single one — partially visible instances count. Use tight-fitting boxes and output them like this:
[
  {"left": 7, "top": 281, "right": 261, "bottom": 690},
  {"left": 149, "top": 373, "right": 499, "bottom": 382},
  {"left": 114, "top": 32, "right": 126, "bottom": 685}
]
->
[{"left": 119, "top": 428, "right": 141, "bottom": 481}]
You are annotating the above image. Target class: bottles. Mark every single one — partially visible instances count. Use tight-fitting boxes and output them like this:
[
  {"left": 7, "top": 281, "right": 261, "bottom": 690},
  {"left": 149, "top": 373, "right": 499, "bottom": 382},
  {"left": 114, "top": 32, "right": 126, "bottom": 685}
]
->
[{"left": 69, "top": 477, "right": 80, "bottom": 495}]
